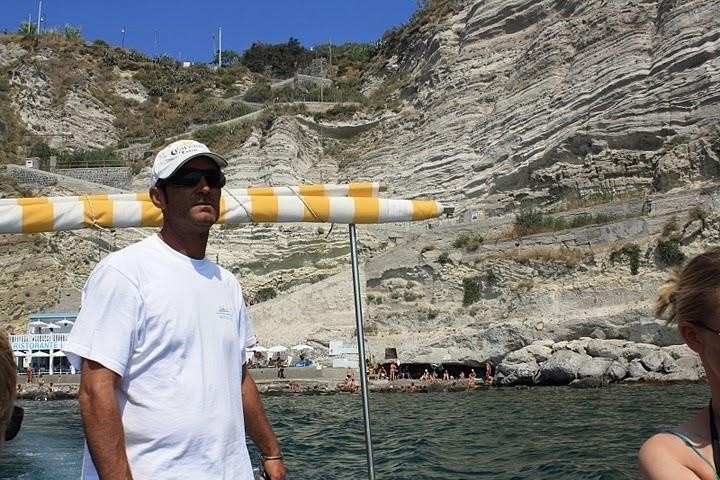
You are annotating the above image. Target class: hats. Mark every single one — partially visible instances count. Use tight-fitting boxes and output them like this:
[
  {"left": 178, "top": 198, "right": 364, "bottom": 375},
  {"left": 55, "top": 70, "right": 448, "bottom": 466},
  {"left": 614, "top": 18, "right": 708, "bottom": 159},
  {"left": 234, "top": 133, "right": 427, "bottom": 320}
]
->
[{"left": 151, "top": 139, "right": 228, "bottom": 187}]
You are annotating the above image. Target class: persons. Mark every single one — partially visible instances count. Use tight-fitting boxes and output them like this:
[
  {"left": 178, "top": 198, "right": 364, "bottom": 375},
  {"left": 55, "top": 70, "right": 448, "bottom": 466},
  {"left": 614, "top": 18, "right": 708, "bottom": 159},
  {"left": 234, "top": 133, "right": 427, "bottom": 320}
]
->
[
  {"left": 61, "top": 139, "right": 288, "bottom": 480},
  {"left": 0, "top": 328, "right": 23, "bottom": 459},
  {"left": 17, "top": 363, "right": 57, "bottom": 399},
  {"left": 639, "top": 246, "right": 720, "bottom": 480},
  {"left": 247, "top": 349, "right": 493, "bottom": 394}
]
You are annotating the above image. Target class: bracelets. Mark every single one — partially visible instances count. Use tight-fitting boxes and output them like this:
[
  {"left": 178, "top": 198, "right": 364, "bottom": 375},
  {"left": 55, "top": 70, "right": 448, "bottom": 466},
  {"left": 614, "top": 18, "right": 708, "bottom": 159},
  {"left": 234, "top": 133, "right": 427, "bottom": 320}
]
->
[{"left": 261, "top": 455, "right": 282, "bottom": 462}]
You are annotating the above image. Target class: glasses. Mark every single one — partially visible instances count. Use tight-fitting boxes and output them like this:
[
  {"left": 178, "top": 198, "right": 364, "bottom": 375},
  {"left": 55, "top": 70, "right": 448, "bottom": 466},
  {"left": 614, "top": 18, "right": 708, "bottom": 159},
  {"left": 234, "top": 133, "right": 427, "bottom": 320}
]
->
[
  {"left": 171, "top": 168, "right": 226, "bottom": 188},
  {"left": 687, "top": 321, "right": 720, "bottom": 337},
  {"left": 4, "top": 406, "right": 24, "bottom": 441}
]
